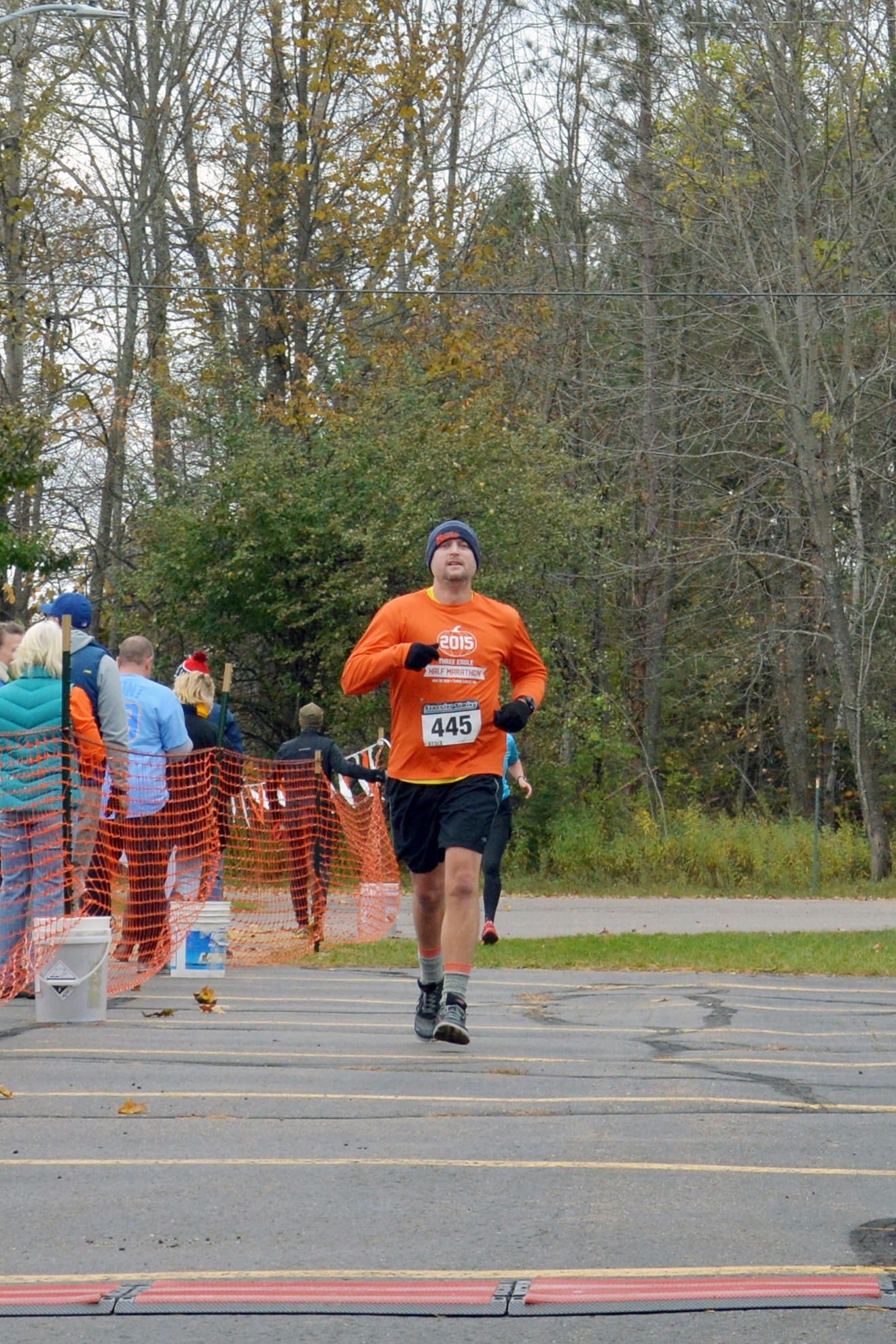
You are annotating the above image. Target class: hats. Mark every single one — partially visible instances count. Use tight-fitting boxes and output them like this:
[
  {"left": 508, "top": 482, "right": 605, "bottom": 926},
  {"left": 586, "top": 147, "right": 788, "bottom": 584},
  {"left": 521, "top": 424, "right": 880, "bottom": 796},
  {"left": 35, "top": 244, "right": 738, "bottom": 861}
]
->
[
  {"left": 41, "top": 592, "right": 91, "bottom": 629},
  {"left": 425, "top": 519, "right": 480, "bottom": 575},
  {"left": 298, "top": 703, "right": 323, "bottom": 729},
  {"left": 174, "top": 650, "right": 209, "bottom": 678}
]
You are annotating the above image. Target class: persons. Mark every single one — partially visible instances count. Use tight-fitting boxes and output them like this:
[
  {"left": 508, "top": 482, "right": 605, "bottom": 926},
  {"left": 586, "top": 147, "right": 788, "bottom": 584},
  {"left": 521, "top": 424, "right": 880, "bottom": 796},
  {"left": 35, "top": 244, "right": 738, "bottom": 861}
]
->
[
  {"left": 264, "top": 702, "right": 388, "bottom": 952},
  {"left": 0, "top": 593, "right": 246, "bottom": 999},
  {"left": 480, "top": 733, "right": 533, "bottom": 945},
  {"left": 340, "top": 523, "right": 548, "bottom": 1045}
]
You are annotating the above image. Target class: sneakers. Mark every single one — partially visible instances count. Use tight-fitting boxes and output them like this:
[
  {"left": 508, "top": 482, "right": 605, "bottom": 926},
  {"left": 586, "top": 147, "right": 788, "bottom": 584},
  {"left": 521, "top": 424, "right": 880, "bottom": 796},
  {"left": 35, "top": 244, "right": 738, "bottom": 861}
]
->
[
  {"left": 414, "top": 978, "right": 443, "bottom": 1040},
  {"left": 433, "top": 994, "right": 471, "bottom": 1045},
  {"left": 481, "top": 923, "right": 499, "bottom": 944}
]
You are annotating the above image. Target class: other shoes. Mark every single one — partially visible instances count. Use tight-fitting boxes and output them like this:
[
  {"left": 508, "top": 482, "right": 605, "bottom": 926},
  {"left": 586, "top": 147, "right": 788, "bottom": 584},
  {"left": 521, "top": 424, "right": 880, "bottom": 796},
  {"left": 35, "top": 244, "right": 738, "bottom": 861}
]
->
[
  {"left": 292, "top": 926, "right": 310, "bottom": 939},
  {"left": 114, "top": 937, "right": 133, "bottom": 961},
  {"left": 11, "top": 980, "right": 34, "bottom": 998},
  {"left": 135, "top": 960, "right": 168, "bottom": 975}
]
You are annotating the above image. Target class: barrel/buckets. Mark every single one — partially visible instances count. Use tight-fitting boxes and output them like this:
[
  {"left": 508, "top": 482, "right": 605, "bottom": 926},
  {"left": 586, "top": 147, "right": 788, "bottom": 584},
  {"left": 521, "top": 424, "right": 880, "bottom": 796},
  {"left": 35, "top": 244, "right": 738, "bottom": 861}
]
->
[
  {"left": 169, "top": 900, "right": 231, "bottom": 978},
  {"left": 33, "top": 916, "right": 111, "bottom": 1022},
  {"left": 361, "top": 883, "right": 399, "bottom": 938}
]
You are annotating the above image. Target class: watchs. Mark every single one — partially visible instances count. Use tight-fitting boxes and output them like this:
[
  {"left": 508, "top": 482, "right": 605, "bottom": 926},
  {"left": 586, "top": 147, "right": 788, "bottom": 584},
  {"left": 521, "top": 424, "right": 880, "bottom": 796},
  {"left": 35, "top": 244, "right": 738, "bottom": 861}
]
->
[{"left": 522, "top": 696, "right": 535, "bottom": 712}]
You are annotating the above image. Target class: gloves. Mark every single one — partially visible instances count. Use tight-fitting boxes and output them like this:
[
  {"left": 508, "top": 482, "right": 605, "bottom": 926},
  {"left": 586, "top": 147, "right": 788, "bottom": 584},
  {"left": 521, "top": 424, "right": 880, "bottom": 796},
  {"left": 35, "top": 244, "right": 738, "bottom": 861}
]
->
[
  {"left": 370, "top": 769, "right": 384, "bottom": 783},
  {"left": 404, "top": 642, "right": 440, "bottom": 672},
  {"left": 493, "top": 700, "right": 530, "bottom": 734}
]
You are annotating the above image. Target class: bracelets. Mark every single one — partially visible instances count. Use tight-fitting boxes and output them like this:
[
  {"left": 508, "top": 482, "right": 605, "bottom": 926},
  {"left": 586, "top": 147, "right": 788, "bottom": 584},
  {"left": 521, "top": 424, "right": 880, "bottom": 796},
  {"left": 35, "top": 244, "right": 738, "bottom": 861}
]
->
[{"left": 517, "top": 776, "right": 527, "bottom": 785}]
